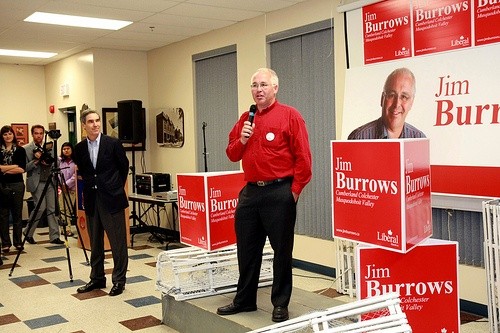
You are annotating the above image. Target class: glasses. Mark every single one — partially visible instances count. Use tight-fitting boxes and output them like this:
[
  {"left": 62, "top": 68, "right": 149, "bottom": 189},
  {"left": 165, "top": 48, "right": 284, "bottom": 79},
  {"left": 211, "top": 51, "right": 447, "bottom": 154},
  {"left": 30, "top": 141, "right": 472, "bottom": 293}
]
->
[
  {"left": 251, "top": 84, "right": 272, "bottom": 89},
  {"left": 383, "top": 91, "right": 413, "bottom": 102}
]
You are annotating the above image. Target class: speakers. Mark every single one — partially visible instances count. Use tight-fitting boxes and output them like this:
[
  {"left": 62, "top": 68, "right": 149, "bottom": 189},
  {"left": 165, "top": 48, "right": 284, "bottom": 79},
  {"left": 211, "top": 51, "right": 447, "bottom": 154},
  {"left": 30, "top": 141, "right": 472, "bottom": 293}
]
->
[{"left": 117, "top": 99, "right": 144, "bottom": 143}]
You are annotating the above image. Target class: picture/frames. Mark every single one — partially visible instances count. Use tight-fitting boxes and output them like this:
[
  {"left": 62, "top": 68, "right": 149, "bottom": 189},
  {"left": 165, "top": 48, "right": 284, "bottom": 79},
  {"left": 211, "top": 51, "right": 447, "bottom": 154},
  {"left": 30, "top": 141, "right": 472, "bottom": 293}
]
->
[
  {"left": 101, "top": 106, "right": 147, "bottom": 152},
  {"left": 10, "top": 123, "right": 29, "bottom": 147}
]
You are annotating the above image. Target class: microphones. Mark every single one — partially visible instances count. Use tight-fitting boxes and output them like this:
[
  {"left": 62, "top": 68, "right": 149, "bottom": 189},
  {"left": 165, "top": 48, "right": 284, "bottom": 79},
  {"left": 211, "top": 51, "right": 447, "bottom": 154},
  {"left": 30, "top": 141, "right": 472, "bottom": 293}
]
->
[{"left": 244, "top": 105, "right": 256, "bottom": 138}]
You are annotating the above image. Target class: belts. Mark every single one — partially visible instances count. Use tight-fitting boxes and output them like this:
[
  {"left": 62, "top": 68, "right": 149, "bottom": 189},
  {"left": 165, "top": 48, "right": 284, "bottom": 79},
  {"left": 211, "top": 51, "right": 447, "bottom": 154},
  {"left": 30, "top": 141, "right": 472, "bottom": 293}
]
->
[{"left": 247, "top": 178, "right": 288, "bottom": 187}]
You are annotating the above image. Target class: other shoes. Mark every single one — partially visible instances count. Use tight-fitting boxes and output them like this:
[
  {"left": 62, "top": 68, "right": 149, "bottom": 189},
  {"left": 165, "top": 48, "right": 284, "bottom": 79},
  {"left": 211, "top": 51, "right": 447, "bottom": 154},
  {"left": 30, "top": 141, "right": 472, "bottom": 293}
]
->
[
  {"left": 61, "top": 231, "right": 74, "bottom": 236},
  {"left": 23, "top": 232, "right": 37, "bottom": 244},
  {"left": 16, "top": 245, "right": 24, "bottom": 250},
  {"left": 3, "top": 247, "right": 9, "bottom": 253},
  {"left": 50, "top": 237, "right": 65, "bottom": 244}
]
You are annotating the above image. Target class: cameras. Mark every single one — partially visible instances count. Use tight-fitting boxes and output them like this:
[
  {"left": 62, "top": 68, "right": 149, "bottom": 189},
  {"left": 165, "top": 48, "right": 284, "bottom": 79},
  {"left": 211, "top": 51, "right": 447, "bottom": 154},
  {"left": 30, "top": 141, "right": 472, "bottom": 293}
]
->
[
  {"left": 34, "top": 146, "right": 54, "bottom": 165},
  {"left": 48, "top": 129, "right": 61, "bottom": 139}
]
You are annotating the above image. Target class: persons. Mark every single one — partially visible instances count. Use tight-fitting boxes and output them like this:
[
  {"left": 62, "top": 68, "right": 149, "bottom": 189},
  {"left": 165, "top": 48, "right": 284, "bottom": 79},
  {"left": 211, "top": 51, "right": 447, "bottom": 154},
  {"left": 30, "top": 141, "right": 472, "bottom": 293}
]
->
[
  {"left": 348, "top": 67, "right": 427, "bottom": 140},
  {"left": 218, "top": 69, "right": 313, "bottom": 324},
  {"left": 0, "top": 125, "right": 76, "bottom": 254},
  {"left": 71, "top": 108, "right": 129, "bottom": 297}
]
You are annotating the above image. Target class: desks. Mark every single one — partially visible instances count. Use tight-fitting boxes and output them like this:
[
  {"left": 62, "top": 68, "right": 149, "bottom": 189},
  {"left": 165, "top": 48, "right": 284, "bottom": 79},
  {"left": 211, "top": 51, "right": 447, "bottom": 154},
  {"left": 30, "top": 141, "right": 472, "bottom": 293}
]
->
[{"left": 128, "top": 191, "right": 178, "bottom": 251}]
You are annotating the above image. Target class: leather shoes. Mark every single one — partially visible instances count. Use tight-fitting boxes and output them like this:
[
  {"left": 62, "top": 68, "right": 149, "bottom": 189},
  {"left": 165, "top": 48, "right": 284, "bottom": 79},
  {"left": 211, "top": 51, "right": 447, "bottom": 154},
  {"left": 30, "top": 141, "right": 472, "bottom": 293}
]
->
[
  {"left": 272, "top": 306, "right": 288, "bottom": 322},
  {"left": 216, "top": 303, "right": 257, "bottom": 314},
  {"left": 77, "top": 278, "right": 106, "bottom": 293},
  {"left": 109, "top": 283, "right": 125, "bottom": 296}
]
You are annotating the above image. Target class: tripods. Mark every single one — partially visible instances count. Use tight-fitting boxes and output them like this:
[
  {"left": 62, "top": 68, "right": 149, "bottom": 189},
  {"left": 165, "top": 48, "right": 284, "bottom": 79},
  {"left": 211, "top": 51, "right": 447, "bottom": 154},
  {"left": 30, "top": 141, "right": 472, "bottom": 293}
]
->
[
  {"left": 128, "top": 143, "right": 165, "bottom": 247},
  {"left": 8, "top": 166, "right": 90, "bottom": 282}
]
